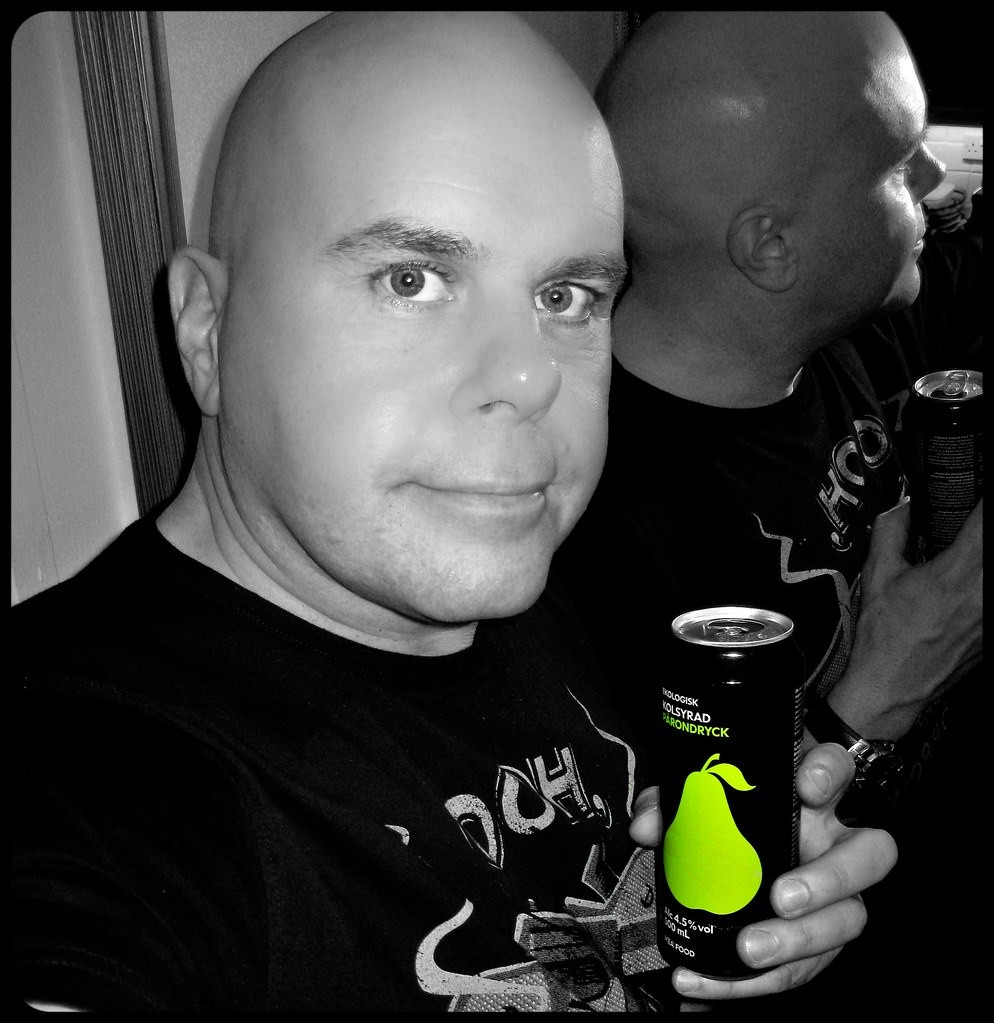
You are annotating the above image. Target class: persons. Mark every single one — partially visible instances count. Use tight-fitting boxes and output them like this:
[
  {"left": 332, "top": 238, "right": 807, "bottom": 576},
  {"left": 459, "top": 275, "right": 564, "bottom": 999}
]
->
[
  {"left": 10, "top": 12, "right": 902, "bottom": 1014},
  {"left": 543, "top": 12, "right": 982, "bottom": 832}
]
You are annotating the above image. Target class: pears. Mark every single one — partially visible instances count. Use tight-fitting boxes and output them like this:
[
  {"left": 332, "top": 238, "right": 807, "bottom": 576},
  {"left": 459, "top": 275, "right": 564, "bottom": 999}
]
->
[{"left": 662, "top": 752, "right": 763, "bottom": 918}]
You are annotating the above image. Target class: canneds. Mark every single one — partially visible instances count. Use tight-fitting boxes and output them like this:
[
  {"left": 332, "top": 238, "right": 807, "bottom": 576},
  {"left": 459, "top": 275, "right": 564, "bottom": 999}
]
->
[
  {"left": 656, "top": 603, "right": 804, "bottom": 983},
  {"left": 900, "top": 369, "right": 982, "bottom": 566}
]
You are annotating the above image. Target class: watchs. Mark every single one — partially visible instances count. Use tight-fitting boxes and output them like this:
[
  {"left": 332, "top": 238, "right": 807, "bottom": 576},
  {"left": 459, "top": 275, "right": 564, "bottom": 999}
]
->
[{"left": 802, "top": 692, "right": 909, "bottom": 805}]
